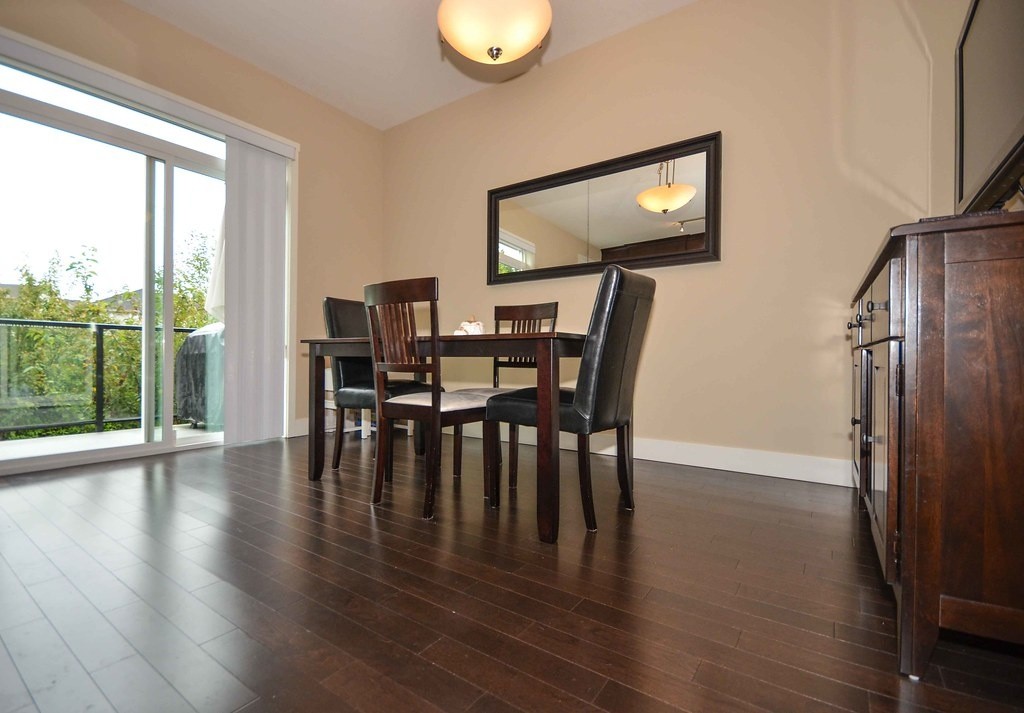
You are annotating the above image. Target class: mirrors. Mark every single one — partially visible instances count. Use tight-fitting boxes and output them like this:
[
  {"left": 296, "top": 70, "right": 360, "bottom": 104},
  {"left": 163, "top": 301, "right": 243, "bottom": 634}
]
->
[{"left": 486, "top": 129, "right": 722, "bottom": 285}]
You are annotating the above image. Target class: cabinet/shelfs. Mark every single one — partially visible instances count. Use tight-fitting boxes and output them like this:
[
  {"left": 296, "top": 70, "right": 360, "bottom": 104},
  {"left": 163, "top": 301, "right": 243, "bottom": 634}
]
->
[{"left": 848, "top": 209, "right": 1024, "bottom": 679}]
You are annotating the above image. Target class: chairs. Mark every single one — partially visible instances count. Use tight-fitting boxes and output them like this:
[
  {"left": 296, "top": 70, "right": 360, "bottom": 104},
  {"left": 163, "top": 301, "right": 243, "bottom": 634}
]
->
[
  {"left": 494, "top": 264, "right": 656, "bottom": 532},
  {"left": 363, "top": 275, "right": 490, "bottom": 520},
  {"left": 323, "top": 296, "right": 446, "bottom": 484},
  {"left": 451, "top": 301, "right": 559, "bottom": 489}
]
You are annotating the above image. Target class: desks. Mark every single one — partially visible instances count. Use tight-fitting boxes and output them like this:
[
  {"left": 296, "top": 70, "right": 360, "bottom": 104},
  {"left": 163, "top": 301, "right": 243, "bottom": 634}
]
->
[
  {"left": 175, "top": 321, "right": 224, "bottom": 429},
  {"left": 300, "top": 332, "right": 586, "bottom": 543}
]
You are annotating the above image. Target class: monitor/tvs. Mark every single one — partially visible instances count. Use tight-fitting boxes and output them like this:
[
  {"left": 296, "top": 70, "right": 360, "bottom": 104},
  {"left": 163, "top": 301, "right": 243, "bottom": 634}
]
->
[{"left": 954, "top": 0, "right": 1024, "bottom": 217}]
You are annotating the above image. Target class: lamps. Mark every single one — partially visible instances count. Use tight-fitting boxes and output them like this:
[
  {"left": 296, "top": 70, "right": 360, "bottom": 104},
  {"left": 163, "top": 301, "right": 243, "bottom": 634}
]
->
[
  {"left": 636, "top": 159, "right": 696, "bottom": 214},
  {"left": 437, "top": 0, "right": 552, "bottom": 66}
]
